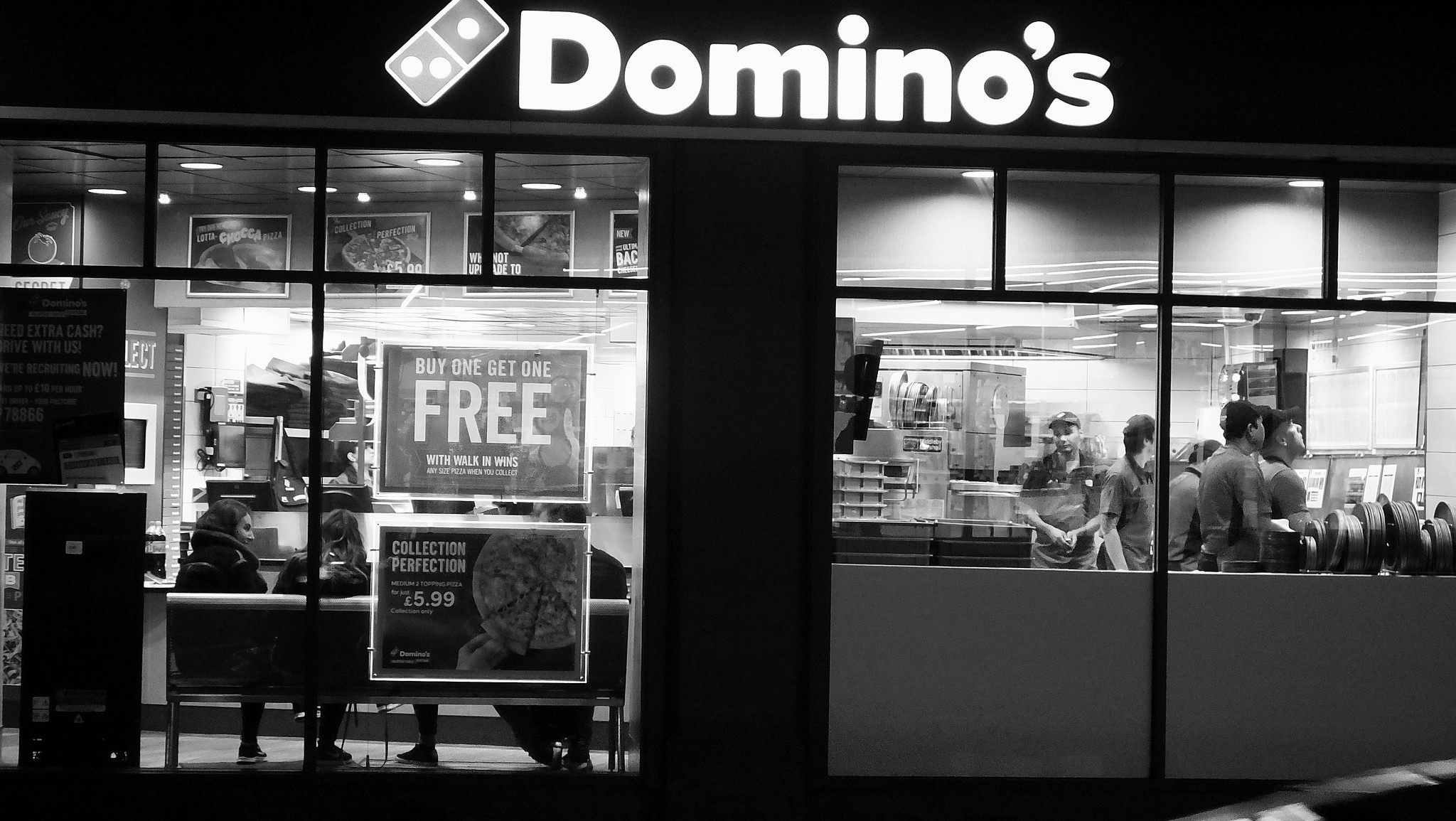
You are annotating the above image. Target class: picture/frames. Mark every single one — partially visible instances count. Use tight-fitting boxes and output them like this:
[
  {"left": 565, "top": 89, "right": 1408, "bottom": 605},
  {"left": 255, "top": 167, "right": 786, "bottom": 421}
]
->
[
  {"left": 185, "top": 213, "right": 293, "bottom": 299},
  {"left": 323, "top": 212, "right": 432, "bottom": 298},
  {"left": 608, "top": 209, "right": 638, "bottom": 299},
  {"left": 463, "top": 210, "right": 575, "bottom": 297}
]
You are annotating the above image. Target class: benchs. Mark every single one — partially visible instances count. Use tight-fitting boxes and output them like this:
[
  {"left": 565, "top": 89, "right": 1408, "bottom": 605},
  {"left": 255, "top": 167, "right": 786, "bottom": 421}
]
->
[{"left": 165, "top": 592, "right": 629, "bottom": 772}]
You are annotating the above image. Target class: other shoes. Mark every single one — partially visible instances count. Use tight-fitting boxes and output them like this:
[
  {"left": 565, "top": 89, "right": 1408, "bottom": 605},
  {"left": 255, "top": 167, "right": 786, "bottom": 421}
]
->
[
  {"left": 238, "top": 743, "right": 267, "bottom": 762},
  {"left": 316, "top": 744, "right": 352, "bottom": 766},
  {"left": 292, "top": 702, "right": 321, "bottom": 722},
  {"left": 396, "top": 743, "right": 439, "bottom": 766},
  {"left": 549, "top": 741, "right": 562, "bottom": 768},
  {"left": 561, "top": 754, "right": 594, "bottom": 772}
]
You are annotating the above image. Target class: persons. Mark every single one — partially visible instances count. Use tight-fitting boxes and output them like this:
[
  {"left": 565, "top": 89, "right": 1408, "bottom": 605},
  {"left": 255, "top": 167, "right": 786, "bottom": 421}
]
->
[
  {"left": 393, "top": 502, "right": 628, "bottom": 770},
  {"left": 271, "top": 508, "right": 405, "bottom": 767},
  {"left": 1166, "top": 440, "right": 1223, "bottom": 571},
  {"left": 1196, "top": 400, "right": 1272, "bottom": 572},
  {"left": 456, "top": 633, "right": 509, "bottom": 673},
  {"left": 1017, "top": 411, "right": 1102, "bottom": 570},
  {"left": 328, "top": 441, "right": 374, "bottom": 498},
  {"left": 1256, "top": 405, "right": 1312, "bottom": 536},
  {"left": 174, "top": 498, "right": 269, "bottom": 763},
  {"left": 1097, "top": 414, "right": 1155, "bottom": 571}
]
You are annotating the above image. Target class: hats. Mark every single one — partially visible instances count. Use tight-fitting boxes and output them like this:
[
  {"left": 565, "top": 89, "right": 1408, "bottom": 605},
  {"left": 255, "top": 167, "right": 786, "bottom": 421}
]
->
[
  {"left": 1193, "top": 440, "right": 1222, "bottom": 453},
  {"left": 1049, "top": 412, "right": 1081, "bottom": 432},
  {"left": 1220, "top": 402, "right": 1272, "bottom": 430},
  {"left": 1123, "top": 414, "right": 1156, "bottom": 438},
  {"left": 337, "top": 441, "right": 374, "bottom": 460},
  {"left": 1273, "top": 406, "right": 1300, "bottom": 426}
]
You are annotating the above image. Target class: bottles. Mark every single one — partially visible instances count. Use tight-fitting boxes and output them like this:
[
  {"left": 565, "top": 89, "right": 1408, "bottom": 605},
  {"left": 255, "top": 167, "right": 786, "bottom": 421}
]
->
[
  {"left": 151, "top": 520, "right": 166, "bottom": 580},
  {"left": 144, "top": 520, "right": 155, "bottom": 575}
]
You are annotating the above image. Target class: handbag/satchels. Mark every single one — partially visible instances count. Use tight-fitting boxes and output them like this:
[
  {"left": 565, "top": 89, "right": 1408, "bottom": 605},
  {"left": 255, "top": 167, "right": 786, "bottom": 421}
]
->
[{"left": 271, "top": 475, "right": 309, "bottom": 512}]
